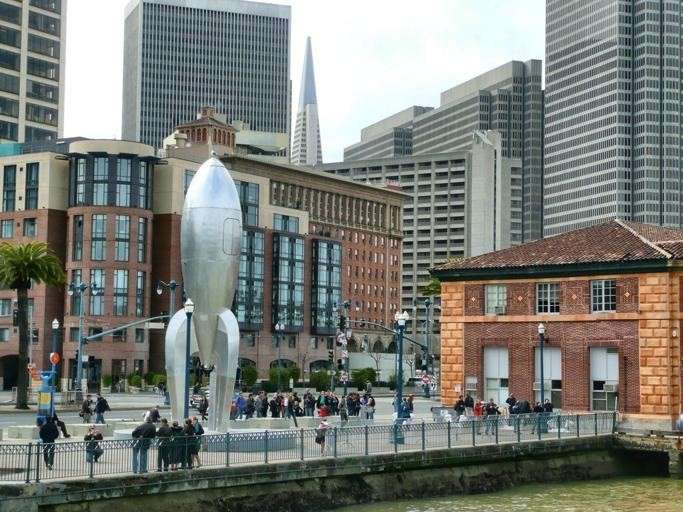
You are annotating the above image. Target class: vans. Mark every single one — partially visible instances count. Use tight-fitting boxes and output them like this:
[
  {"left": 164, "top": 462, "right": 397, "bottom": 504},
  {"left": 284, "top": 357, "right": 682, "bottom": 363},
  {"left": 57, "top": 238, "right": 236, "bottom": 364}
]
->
[{"left": 408, "top": 377, "right": 422, "bottom": 385}]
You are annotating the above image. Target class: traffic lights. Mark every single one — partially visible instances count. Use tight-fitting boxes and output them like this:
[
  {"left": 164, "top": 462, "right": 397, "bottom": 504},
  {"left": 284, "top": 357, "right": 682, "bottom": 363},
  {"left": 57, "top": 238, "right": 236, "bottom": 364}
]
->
[
  {"left": 342, "top": 317, "right": 346, "bottom": 331},
  {"left": 328, "top": 351, "right": 333, "bottom": 364},
  {"left": 337, "top": 317, "right": 341, "bottom": 332}
]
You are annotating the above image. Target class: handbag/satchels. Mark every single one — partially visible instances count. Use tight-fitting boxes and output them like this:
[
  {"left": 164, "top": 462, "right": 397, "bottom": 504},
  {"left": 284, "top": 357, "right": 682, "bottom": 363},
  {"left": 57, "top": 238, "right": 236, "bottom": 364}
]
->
[{"left": 315, "top": 437, "right": 321, "bottom": 444}]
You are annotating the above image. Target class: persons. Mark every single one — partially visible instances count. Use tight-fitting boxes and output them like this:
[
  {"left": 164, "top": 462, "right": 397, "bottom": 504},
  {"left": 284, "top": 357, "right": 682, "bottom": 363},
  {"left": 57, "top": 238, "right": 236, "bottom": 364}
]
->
[
  {"left": 190, "top": 416, "right": 205, "bottom": 470},
  {"left": 315, "top": 418, "right": 329, "bottom": 457},
  {"left": 452, "top": 392, "right": 554, "bottom": 436},
  {"left": 31, "top": 416, "right": 44, "bottom": 443},
  {"left": 84, "top": 424, "right": 105, "bottom": 477},
  {"left": 155, "top": 417, "right": 173, "bottom": 472},
  {"left": 169, "top": 420, "right": 184, "bottom": 470},
  {"left": 53, "top": 407, "right": 71, "bottom": 438},
  {"left": 130, "top": 415, "right": 157, "bottom": 473},
  {"left": 391, "top": 377, "right": 434, "bottom": 419},
  {"left": 230, "top": 380, "right": 376, "bottom": 428},
  {"left": 144, "top": 378, "right": 209, "bottom": 423},
  {"left": 38, "top": 415, "right": 59, "bottom": 471},
  {"left": 81, "top": 393, "right": 106, "bottom": 424},
  {"left": 181, "top": 418, "right": 196, "bottom": 469}
]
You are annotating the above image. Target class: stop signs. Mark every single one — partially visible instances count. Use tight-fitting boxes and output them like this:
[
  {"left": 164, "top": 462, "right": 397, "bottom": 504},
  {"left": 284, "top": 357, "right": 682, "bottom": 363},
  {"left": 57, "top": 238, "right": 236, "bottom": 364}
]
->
[
  {"left": 341, "top": 372, "right": 348, "bottom": 381},
  {"left": 422, "top": 376, "right": 429, "bottom": 384}
]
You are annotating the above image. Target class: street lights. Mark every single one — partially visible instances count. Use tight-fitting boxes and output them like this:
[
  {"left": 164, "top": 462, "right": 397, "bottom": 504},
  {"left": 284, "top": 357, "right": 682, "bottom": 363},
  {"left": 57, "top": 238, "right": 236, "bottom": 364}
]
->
[
  {"left": 49, "top": 317, "right": 60, "bottom": 423},
  {"left": 341, "top": 301, "right": 350, "bottom": 396},
  {"left": 156, "top": 280, "right": 179, "bottom": 321},
  {"left": 275, "top": 322, "right": 285, "bottom": 393},
  {"left": 422, "top": 299, "right": 432, "bottom": 398},
  {"left": 391, "top": 311, "right": 409, "bottom": 445},
  {"left": 184, "top": 298, "right": 195, "bottom": 425},
  {"left": 66, "top": 282, "right": 97, "bottom": 392},
  {"left": 537, "top": 322, "right": 548, "bottom": 435}
]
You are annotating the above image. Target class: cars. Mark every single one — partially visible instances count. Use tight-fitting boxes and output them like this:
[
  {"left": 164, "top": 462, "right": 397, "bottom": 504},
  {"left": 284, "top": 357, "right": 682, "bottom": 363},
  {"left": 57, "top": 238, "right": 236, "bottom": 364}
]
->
[{"left": 190, "top": 389, "right": 211, "bottom": 407}]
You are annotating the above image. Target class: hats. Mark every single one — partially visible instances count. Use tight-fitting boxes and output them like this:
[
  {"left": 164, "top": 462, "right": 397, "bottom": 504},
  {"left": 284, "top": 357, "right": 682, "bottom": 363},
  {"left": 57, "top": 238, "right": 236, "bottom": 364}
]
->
[
  {"left": 144, "top": 416, "right": 152, "bottom": 423},
  {"left": 321, "top": 420, "right": 328, "bottom": 425}
]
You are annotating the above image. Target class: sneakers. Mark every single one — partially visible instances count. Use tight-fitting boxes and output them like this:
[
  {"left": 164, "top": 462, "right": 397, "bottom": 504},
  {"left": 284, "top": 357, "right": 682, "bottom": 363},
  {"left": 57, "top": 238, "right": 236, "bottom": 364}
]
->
[{"left": 48, "top": 464, "right": 52, "bottom": 470}]
